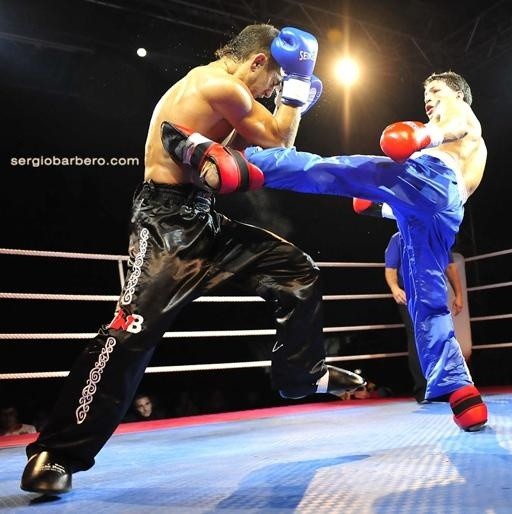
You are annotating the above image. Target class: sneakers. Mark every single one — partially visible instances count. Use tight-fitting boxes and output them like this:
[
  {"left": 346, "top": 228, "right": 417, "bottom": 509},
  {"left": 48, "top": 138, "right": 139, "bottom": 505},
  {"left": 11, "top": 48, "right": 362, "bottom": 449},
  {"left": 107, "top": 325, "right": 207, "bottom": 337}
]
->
[
  {"left": 160, "top": 120, "right": 267, "bottom": 195},
  {"left": 448, "top": 385, "right": 491, "bottom": 433},
  {"left": 279, "top": 364, "right": 367, "bottom": 400},
  {"left": 20, "top": 451, "right": 73, "bottom": 494}
]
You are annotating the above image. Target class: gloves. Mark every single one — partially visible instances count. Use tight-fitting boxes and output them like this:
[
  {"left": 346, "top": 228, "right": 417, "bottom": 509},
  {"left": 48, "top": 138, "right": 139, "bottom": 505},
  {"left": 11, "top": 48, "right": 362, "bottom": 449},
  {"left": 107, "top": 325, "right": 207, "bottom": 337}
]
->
[
  {"left": 271, "top": 27, "right": 320, "bottom": 107},
  {"left": 278, "top": 71, "right": 324, "bottom": 117},
  {"left": 379, "top": 118, "right": 441, "bottom": 165},
  {"left": 353, "top": 194, "right": 399, "bottom": 221}
]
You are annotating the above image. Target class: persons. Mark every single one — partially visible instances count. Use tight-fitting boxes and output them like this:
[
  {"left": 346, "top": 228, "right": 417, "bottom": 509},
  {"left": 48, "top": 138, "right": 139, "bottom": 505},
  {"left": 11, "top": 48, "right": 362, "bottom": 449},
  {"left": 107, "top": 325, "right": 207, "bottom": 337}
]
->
[
  {"left": 133, "top": 393, "right": 159, "bottom": 422},
  {"left": 1, "top": 401, "right": 37, "bottom": 437},
  {"left": 384, "top": 224, "right": 464, "bottom": 406},
  {"left": 340, "top": 381, "right": 392, "bottom": 400},
  {"left": 19, "top": 21, "right": 369, "bottom": 498},
  {"left": 161, "top": 68, "right": 488, "bottom": 434}
]
